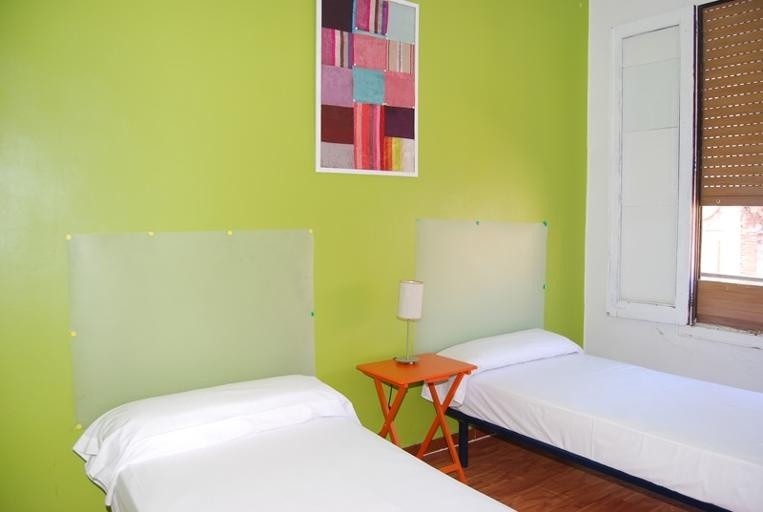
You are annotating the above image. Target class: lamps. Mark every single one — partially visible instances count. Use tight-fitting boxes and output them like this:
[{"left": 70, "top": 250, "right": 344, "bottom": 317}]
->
[{"left": 392, "top": 280, "right": 423, "bottom": 365}]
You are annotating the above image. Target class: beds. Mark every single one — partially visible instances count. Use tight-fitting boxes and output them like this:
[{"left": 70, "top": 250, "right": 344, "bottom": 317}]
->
[
  {"left": 93, "top": 371, "right": 518, "bottom": 512},
  {"left": 425, "top": 329, "right": 763, "bottom": 511}
]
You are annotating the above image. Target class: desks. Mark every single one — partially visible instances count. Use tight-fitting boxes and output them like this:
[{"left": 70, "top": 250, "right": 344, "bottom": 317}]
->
[{"left": 355, "top": 353, "right": 477, "bottom": 483}]
